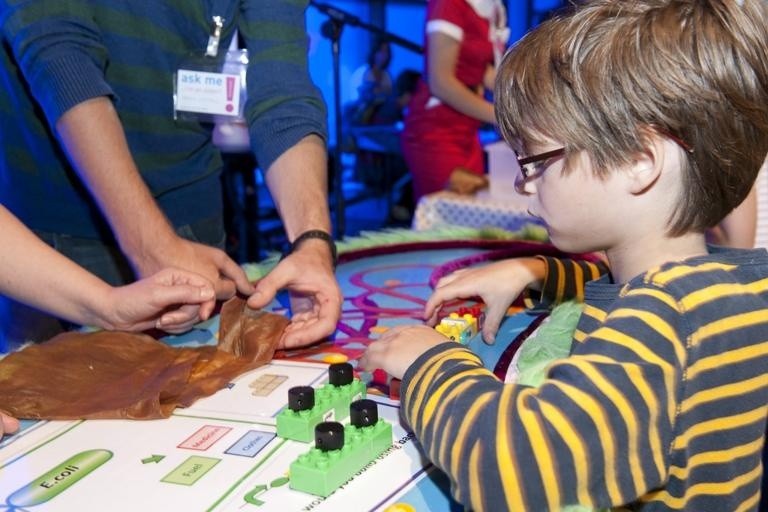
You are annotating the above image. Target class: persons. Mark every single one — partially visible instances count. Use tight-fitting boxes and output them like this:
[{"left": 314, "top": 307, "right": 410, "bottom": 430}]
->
[
  {"left": 350, "top": 35, "right": 392, "bottom": 105},
  {"left": 354, "top": 70, "right": 425, "bottom": 220},
  {"left": 402, "top": 1, "right": 510, "bottom": 202},
  {"left": 0, "top": 202, "right": 216, "bottom": 443},
  {"left": 356, "top": 2, "right": 767, "bottom": 512},
  {"left": 2, "top": 2, "right": 344, "bottom": 350}
]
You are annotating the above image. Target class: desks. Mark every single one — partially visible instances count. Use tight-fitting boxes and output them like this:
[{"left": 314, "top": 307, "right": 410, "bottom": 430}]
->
[{"left": 1, "top": 173, "right": 603, "bottom": 510}]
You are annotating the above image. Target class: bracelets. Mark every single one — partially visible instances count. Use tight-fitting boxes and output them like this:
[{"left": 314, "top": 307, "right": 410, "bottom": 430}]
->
[{"left": 291, "top": 228, "right": 337, "bottom": 269}]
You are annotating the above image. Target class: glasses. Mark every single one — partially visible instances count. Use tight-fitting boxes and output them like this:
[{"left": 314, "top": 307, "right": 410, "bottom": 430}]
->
[{"left": 515, "top": 146, "right": 565, "bottom": 182}]
